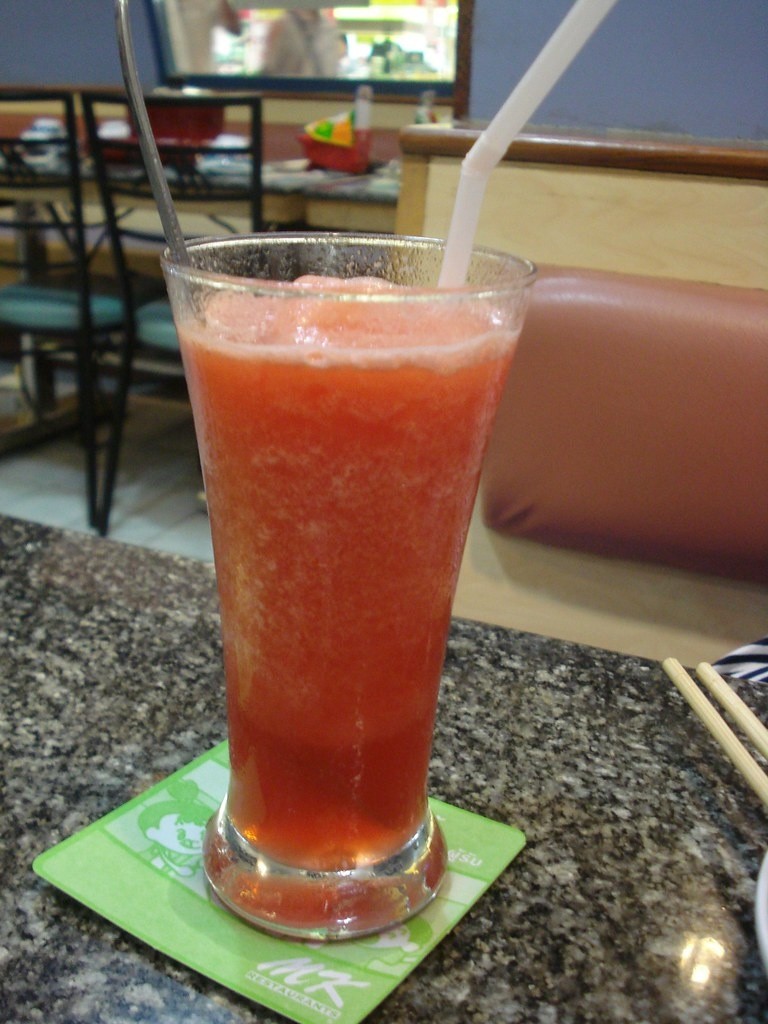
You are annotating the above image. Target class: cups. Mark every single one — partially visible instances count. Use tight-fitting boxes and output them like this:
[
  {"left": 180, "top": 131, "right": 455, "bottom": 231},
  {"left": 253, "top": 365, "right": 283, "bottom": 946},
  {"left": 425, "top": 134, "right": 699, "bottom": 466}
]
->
[{"left": 159, "top": 232, "right": 539, "bottom": 942}]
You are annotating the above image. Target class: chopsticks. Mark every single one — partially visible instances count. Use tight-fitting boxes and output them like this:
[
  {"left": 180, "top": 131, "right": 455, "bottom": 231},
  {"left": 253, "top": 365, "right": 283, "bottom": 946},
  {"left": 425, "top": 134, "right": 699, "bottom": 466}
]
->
[{"left": 662, "top": 656, "right": 768, "bottom": 809}]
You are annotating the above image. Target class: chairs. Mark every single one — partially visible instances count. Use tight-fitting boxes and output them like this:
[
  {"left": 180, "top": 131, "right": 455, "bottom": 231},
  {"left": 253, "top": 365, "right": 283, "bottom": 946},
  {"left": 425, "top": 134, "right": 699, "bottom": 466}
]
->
[{"left": 1, "top": 85, "right": 275, "bottom": 537}]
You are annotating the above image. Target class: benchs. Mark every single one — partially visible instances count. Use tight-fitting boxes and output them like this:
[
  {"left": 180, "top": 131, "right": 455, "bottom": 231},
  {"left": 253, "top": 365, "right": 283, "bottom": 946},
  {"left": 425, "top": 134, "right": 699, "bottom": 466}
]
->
[{"left": 388, "top": 122, "right": 768, "bottom": 667}]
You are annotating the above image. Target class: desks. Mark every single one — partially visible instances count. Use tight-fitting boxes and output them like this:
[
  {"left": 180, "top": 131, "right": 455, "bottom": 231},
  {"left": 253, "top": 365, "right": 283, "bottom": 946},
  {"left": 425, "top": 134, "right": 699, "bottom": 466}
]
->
[
  {"left": 0, "top": 148, "right": 401, "bottom": 422},
  {"left": 0, "top": 512, "right": 768, "bottom": 1024}
]
